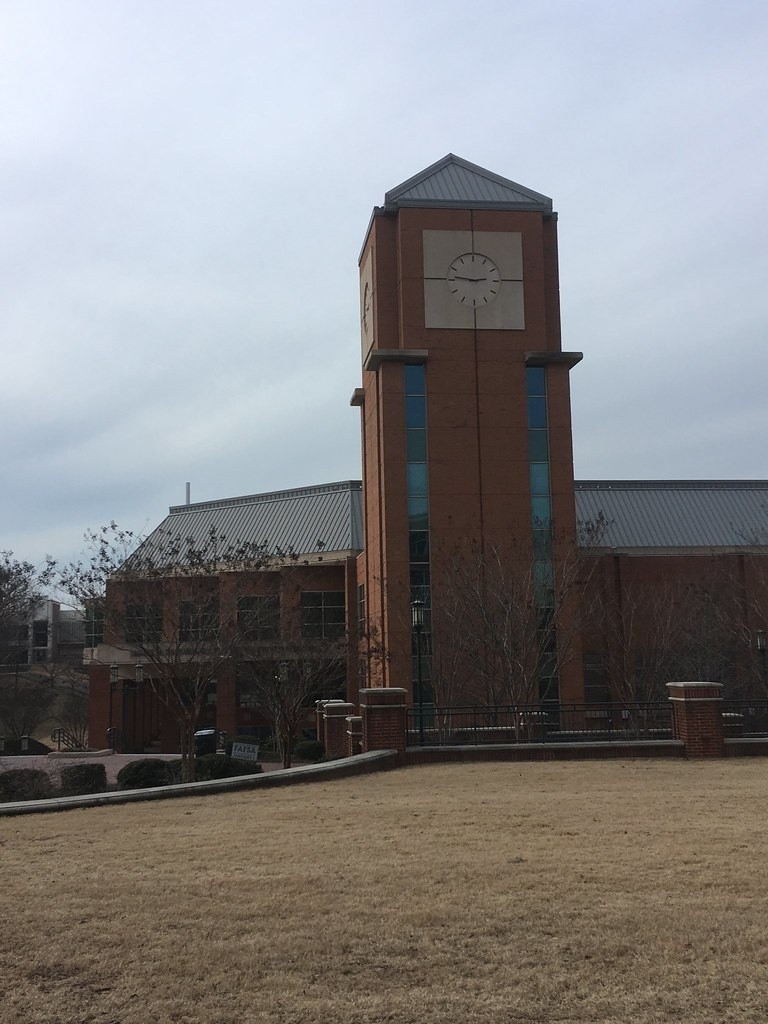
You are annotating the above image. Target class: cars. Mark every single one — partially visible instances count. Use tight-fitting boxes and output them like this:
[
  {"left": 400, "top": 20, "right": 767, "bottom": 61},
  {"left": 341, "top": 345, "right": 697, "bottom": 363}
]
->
[{"left": 0, "top": 734, "right": 55, "bottom": 756}]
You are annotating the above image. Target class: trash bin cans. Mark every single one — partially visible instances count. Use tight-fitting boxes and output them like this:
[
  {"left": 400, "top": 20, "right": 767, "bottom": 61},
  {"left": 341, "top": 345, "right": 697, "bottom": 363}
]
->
[{"left": 194, "top": 729, "right": 216, "bottom": 758}]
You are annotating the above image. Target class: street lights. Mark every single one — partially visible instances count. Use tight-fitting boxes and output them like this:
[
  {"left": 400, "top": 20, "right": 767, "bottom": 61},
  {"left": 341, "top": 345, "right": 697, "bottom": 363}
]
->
[
  {"left": 277, "top": 659, "right": 314, "bottom": 771},
  {"left": 108, "top": 661, "right": 146, "bottom": 754},
  {"left": 410, "top": 598, "right": 426, "bottom": 745}
]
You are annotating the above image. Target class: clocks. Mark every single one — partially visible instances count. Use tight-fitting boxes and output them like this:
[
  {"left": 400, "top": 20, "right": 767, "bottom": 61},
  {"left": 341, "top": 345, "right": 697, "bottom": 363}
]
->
[
  {"left": 446, "top": 252, "right": 501, "bottom": 307},
  {"left": 363, "top": 282, "right": 369, "bottom": 333}
]
